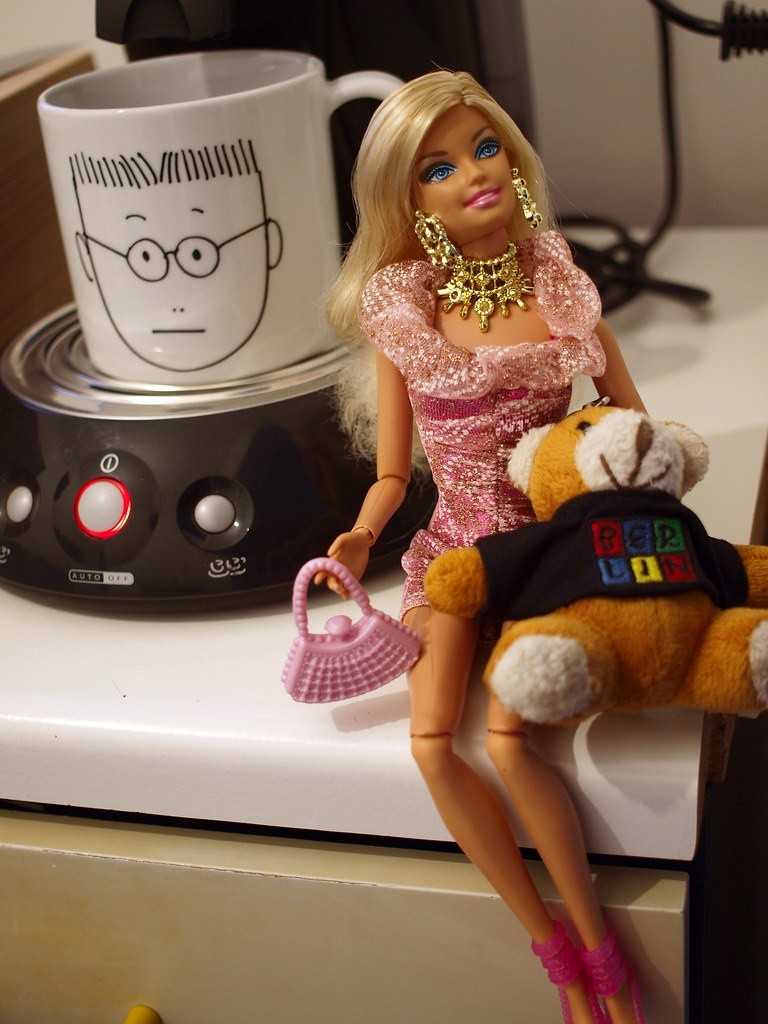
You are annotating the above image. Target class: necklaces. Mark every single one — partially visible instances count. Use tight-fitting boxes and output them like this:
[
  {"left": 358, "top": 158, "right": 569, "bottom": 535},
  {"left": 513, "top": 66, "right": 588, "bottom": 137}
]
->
[{"left": 438, "top": 241, "right": 535, "bottom": 331}]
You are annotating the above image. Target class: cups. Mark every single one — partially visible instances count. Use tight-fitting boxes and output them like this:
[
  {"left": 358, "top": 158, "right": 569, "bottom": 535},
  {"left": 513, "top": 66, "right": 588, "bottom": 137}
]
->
[{"left": 36, "top": 49, "right": 407, "bottom": 388}]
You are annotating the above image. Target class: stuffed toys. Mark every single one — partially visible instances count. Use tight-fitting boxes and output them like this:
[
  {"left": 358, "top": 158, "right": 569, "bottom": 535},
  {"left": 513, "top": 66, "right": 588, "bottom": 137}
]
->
[{"left": 422, "top": 405, "right": 768, "bottom": 727}]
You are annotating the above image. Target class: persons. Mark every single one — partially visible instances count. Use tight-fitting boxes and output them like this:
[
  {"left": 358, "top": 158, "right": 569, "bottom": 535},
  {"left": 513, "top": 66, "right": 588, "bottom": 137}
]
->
[{"left": 315, "top": 71, "right": 651, "bottom": 1024}]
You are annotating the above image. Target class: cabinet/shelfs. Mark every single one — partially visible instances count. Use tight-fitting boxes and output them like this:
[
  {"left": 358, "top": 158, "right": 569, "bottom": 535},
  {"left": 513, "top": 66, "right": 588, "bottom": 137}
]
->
[{"left": 0, "top": 227, "right": 768, "bottom": 1024}]
